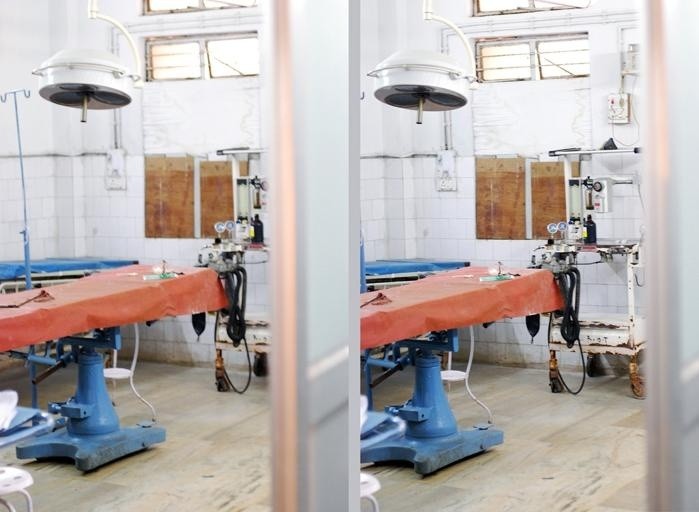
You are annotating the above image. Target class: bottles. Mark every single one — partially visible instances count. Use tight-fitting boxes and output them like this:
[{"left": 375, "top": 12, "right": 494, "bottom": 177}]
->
[
  {"left": 236, "top": 215, "right": 251, "bottom": 242},
  {"left": 584, "top": 214, "right": 597, "bottom": 243},
  {"left": 567, "top": 212, "right": 582, "bottom": 245},
  {"left": 251, "top": 214, "right": 264, "bottom": 243}
]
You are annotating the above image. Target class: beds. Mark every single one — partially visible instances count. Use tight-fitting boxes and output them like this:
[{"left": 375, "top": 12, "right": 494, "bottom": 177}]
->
[
  {"left": 2, "top": 257, "right": 230, "bottom": 468},
  {"left": 359, "top": 255, "right": 568, "bottom": 480}
]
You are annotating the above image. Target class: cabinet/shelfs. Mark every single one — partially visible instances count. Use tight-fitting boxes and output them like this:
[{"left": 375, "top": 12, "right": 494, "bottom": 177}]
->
[
  {"left": 541, "top": 241, "right": 645, "bottom": 399},
  {"left": 200, "top": 240, "right": 272, "bottom": 388}
]
